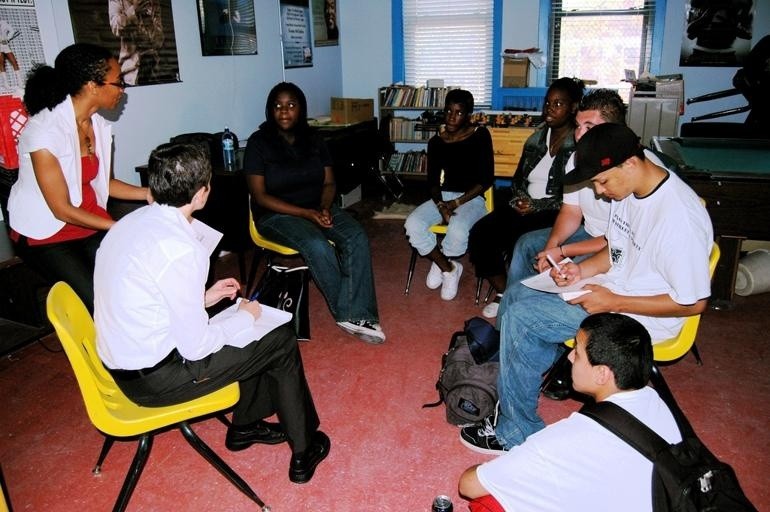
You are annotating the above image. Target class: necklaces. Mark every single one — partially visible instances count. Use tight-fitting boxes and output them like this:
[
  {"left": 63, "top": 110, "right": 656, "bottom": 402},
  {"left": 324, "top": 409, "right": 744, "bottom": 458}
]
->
[{"left": 76, "top": 119, "right": 92, "bottom": 158}]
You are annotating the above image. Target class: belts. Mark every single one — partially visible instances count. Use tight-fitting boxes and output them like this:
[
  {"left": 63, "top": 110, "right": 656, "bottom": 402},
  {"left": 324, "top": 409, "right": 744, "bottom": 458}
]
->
[{"left": 108, "top": 353, "right": 172, "bottom": 380}]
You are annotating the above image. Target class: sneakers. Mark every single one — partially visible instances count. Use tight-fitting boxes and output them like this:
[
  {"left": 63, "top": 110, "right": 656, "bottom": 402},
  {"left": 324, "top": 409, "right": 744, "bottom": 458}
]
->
[
  {"left": 426, "top": 261, "right": 444, "bottom": 290},
  {"left": 337, "top": 319, "right": 386, "bottom": 343},
  {"left": 440, "top": 261, "right": 463, "bottom": 301},
  {"left": 460, "top": 418, "right": 509, "bottom": 456},
  {"left": 483, "top": 293, "right": 503, "bottom": 316}
]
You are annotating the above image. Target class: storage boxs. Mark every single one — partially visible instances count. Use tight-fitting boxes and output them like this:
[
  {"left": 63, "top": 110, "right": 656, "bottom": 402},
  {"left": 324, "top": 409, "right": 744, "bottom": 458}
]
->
[
  {"left": 503, "top": 60, "right": 529, "bottom": 87},
  {"left": 331, "top": 98, "right": 374, "bottom": 124}
]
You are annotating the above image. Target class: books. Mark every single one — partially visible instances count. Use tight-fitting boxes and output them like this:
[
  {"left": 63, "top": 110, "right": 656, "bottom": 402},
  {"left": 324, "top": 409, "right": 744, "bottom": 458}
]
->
[
  {"left": 209, "top": 297, "right": 293, "bottom": 349},
  {"left": 557, "top": 290, "right": 592, "bottom": 301},
  {"left": 379, "top": 86, "right": 454, "bottom": 175}
]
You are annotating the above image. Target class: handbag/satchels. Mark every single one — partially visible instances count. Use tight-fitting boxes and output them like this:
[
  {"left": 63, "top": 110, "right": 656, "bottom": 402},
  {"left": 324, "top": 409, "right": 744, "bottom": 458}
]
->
[
  {"left": 248, "top": 266, "right": 311, "bottom": 342},
  {"left": 437, "top": 331, "right": 500, "bottom": 425}
]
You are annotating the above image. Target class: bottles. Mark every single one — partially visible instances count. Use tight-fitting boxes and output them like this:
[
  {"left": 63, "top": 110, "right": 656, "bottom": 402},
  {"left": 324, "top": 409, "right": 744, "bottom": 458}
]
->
[
  {"left": 431, "top": 494, "right": 454, "bottom": 512},
  {"left": 221, "top": 125, "right": 238, "bottom": 174}
]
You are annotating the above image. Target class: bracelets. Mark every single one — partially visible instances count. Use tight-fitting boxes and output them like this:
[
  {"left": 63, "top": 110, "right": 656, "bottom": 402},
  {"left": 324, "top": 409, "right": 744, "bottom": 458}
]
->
[
  {"left": 455, "top": 199, "right": 460, "bottom": 207},
  {"left": 559, "top": 246, "right": 566, "bottom": 259}
]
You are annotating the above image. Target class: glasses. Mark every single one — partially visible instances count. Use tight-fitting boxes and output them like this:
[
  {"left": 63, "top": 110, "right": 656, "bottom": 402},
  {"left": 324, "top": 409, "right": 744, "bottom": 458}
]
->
[{"left": 104, "top": 78, "right": 127, "bottom": 91}]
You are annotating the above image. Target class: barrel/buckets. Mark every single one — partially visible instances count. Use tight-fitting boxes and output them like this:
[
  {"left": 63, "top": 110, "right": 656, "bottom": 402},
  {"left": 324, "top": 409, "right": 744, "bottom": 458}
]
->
[{"left": 734, "top": 249, "right": 770, "bottom": 297}]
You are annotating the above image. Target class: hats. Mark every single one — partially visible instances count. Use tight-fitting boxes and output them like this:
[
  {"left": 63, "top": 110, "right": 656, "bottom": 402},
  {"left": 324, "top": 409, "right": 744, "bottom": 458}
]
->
[
  {"left": 561, "top": 121, "right": 642, "bottom": 184},
  {"left": 464, "top": 316, "right": 500, "bottom": 364}
]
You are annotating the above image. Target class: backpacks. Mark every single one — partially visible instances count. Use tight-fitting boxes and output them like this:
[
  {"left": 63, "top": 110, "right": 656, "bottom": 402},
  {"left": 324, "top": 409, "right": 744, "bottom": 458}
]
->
[{"left": 578, "top": 400, "right": 757, "bottom": 512}]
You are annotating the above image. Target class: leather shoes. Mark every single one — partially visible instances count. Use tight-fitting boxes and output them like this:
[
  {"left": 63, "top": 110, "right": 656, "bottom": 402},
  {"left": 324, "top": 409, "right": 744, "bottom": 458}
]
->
[
  {"left": 289, "top": 429, "right": 329, "bottom": 483},
  {"left": 225, "top": 421, "right": 285, "bottom": 452}
]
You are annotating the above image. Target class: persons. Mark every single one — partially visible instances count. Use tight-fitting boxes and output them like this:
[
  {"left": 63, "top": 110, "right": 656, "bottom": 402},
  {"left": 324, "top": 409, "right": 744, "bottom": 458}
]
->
[
  {"left": 92, "top": 138, "right": 329, "bottom": 483},
  {"left": 108, "top": 0, "right": 165, "bottom": 84},
  {"left": 0, "top": 16, "right": 24, "bottom": 90},
  {"left": 468, "top": 78, "right": 583, "bottom": 319},
  {"left": 459, "top": 125, "right": 713, "bottom": 454},
  {"left": 405, "top": 89, "right": 494, "bottom": 300},
  {"left": 323, "top": 0, "right": 339, "bottom": 42},
  {"left": 243, "top": 82, "right": 386, "bottom": 345},
  {"left": 494, "top": 87, "right": 662, "bottom": 332},
  {"left": 6, "top": 44, "right": 154, "bottom": 317},
  {"left": 454, "top": 310, "right": 698, "bottom": 512}
]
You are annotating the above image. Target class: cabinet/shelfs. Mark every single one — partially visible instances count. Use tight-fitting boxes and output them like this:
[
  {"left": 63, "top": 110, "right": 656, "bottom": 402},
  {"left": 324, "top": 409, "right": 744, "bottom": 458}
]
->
[{"left": 378, "top": 88, "right": 456, "bottom": 202}]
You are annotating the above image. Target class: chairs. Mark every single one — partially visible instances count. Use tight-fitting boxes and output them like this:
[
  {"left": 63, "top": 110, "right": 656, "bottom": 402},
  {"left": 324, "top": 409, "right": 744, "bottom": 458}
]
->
[
  {"left": 564, "top": 241, "right": 721, "bottom": 366},
  {"left": 46, "top": 282, "right": 266, "bottom": 511},
  {"left": 405, "top": 185, "right": 494, "bottom": 307},
  {"left": 246, "top": 193, "right": 337, "bottom": 298}
]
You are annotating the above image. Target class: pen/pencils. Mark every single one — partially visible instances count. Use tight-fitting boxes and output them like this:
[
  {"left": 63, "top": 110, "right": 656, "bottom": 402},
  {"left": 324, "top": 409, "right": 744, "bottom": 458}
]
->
[
  {"left": 250, "top": 290, "right": 260, "bottom": 301},
  {"left": 546, "top": 254, "right": 567, "bottom": 280}
]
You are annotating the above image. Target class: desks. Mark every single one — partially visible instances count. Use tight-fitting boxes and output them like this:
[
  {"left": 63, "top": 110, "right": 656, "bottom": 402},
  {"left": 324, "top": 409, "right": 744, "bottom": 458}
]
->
[
  {"left": 136, "top": 163, "right": 252, "bottom": 284},
  {"left": 651, "top": 136, "right": 770, "bottom": 311}
]
